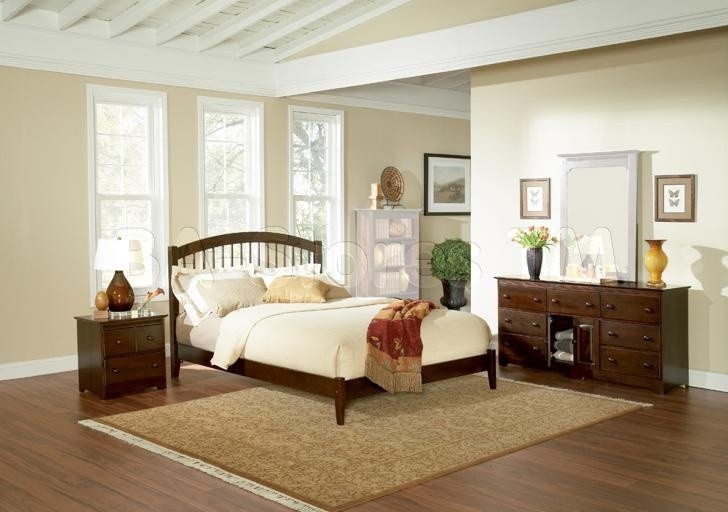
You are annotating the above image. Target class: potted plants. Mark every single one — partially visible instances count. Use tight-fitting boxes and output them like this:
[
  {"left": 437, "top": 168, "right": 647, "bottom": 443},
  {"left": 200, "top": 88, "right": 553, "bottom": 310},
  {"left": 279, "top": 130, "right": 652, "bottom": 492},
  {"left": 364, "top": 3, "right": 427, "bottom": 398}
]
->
[{"left": 427, "top": 238, "right": 471, "bottom": 310}]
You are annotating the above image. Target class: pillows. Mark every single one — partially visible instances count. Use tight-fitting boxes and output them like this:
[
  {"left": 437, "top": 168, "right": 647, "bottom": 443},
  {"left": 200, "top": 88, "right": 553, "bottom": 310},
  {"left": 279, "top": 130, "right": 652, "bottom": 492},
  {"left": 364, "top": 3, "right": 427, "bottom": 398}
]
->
[
  {"left": 171, "top": 264, "right": 267, "bottom": 326},
  {"left": 196, "top": 277, "right": 267, "bottom": 317},
  {"left": 263, "top": 264, "right": 321, "bottom": 290},
  {"left": 315, "top": 275, "right": 351, "bottom": 299},
  {"left": 263, "top": 274, "right": 328, "bottom": 305}
]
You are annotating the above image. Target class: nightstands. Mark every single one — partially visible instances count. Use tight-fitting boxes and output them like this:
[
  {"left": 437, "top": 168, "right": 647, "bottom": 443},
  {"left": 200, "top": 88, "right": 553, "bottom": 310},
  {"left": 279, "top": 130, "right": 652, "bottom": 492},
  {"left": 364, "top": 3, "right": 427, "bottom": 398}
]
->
[{"left": 73, "top": 309, "right": 168, "bottom": 400}]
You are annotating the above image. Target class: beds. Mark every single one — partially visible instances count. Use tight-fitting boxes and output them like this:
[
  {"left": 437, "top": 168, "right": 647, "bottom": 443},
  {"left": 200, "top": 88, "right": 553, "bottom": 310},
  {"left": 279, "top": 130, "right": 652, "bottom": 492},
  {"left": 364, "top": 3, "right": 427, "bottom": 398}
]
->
[{"left": 168, "top": 231, "right": 497, "bottom": 425}]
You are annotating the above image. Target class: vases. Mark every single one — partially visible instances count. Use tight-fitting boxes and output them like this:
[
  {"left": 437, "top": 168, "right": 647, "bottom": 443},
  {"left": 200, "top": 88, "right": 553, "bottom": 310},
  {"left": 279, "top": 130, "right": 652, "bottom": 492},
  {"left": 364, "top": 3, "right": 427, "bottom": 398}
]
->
[
  {"left": 644, "top": 239, "right": 667, "bottom": 286},
  {"left": 527, "top": 247, "right": 543, "bottom": 279}
]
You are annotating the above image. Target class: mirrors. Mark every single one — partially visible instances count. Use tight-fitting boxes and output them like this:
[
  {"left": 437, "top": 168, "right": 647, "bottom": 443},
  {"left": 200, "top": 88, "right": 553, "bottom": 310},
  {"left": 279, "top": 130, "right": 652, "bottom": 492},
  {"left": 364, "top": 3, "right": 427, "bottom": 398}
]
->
[{"left": 557, "top": 148, "right": 639, "bottom": 280}]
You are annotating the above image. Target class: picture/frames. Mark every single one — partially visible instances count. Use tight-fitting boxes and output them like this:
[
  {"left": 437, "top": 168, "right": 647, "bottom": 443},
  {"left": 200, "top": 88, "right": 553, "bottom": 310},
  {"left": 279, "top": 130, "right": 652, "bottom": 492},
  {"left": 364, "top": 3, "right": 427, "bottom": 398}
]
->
[
  {"left": 654, "top": 174, "right": 696, "bottom": 222},
  {"left": 520, "top": 178, "right": 552, "bottom": 220},
  {"left": 423, "top": 152, "right": 471, "bottom": 215}
]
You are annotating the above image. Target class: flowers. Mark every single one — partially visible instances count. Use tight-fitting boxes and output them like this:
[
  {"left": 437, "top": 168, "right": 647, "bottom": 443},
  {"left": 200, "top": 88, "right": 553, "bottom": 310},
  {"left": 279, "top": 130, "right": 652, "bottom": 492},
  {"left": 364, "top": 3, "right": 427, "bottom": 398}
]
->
[{"left": 512, "top": 224, "right": 558, "bottom": 252}]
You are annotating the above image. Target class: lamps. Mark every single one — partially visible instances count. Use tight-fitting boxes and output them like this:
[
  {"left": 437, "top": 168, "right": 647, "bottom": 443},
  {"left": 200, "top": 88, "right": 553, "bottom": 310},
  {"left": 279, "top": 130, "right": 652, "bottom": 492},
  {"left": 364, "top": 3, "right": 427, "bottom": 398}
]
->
[{"left": 93, "top": 237, "right": 144, "bottom": 311}]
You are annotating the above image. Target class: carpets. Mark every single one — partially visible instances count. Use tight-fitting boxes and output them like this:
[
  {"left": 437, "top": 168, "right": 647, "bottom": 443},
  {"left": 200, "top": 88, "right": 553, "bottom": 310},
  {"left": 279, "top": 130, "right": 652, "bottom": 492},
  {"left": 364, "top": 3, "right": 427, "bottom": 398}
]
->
[{"left": 76, "top": 375, "right": 653, "bottom": 512}]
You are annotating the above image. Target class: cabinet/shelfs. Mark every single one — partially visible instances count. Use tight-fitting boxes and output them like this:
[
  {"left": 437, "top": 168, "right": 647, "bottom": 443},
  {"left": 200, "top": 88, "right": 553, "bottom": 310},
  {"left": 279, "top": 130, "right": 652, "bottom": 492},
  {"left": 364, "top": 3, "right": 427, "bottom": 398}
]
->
[
  {"left": 355, "top": 208, "right": 422, "bottom": 301},
  {"left": 493, "top": 274, "right": 692, "bottom": 394}
]
